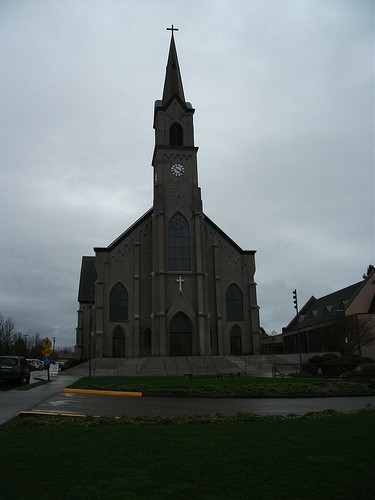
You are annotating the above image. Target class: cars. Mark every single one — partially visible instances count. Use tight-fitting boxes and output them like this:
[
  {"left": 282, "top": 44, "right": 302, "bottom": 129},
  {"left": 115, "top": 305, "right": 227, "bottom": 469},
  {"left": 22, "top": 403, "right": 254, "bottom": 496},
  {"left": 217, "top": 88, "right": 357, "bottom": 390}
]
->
[
  {"left": 25, "top": 357, "right": 70, "bottom": 370},
  {"left": 0, "top": 356, "right": 31, "bottom": 386}
]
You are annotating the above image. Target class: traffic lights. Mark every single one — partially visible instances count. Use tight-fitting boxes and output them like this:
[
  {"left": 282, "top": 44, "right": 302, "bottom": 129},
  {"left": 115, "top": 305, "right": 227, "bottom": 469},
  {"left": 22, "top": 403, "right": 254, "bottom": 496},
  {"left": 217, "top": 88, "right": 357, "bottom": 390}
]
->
[{"left": 293, "top": 290, "right": 297, "bottom": 309}]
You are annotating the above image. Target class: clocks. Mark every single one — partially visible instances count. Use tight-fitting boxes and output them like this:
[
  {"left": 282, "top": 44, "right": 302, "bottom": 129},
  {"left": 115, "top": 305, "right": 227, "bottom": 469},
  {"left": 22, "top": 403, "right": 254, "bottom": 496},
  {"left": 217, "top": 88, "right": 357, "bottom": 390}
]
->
[{"left": 170, "top": 163, "right": 185, "bottom": 177}]
